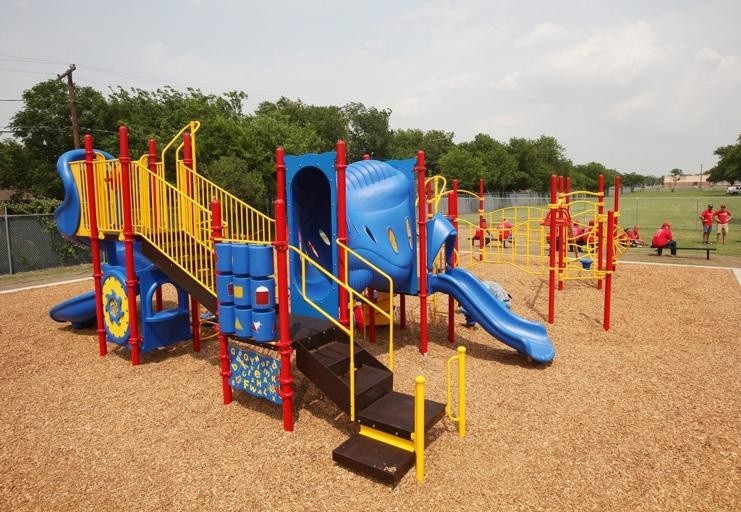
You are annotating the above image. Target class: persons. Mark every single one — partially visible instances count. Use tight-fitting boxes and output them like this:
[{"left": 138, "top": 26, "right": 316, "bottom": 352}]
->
[
  {"left": 476, "top": 220, "right": 494, "bottom": 247},
  {"left": 713, "top": 204, "right": 733, "bottom": 244},
  {"left": 621, "top": 227, "right": 651, "bottom": 247},
  {"left": 652, "top": 222, "right": 678, "bottom": 255},
  {"left": 629, "top": 224, "right": 650, "bottom": 243},
  {"left": 583, "top": 221, "right": 600, "bottom": 249},
  {"left": 570, "top": 219, "right": 583, "bottom": 251},
  {"left": 497, "top": 218, "right": 518, "bottom": 248},
  {"left": 546, "top": 224, "right": 560, "bottom": 249},
  {"left": 699, "top": 203, "right": 716, "bottom": 245}
]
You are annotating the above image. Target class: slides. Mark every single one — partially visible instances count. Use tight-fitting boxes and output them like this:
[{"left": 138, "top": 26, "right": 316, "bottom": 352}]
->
[{"left": 432, "top": 268, "right": 554, "bottom": 364}]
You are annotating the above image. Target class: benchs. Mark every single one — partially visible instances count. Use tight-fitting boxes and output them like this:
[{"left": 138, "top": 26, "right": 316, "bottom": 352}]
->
[
  {"left": 651, "top": 247, "right": 717, "bottom": 260},
  {"left": 468, "top": 238, "right": 512, "bottom": 248},
  {"left": 548, "top": 239, "right": 597, "bottom": 252}
]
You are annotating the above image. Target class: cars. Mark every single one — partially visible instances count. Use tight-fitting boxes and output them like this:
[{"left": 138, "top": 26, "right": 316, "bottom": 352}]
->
[{"left": 727, "top": 185, "right": 741, "bottom": 196}]
